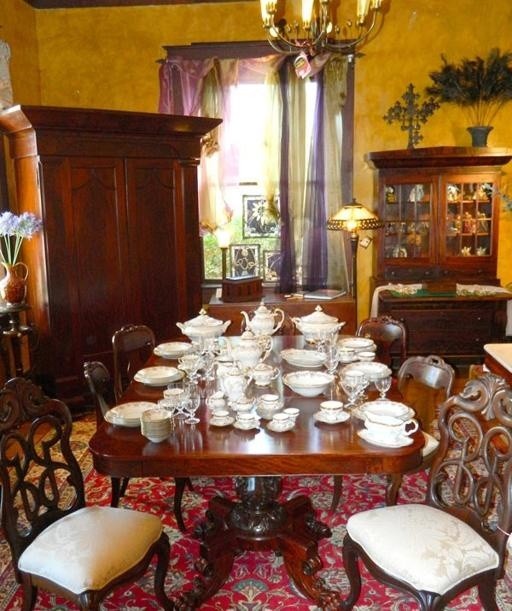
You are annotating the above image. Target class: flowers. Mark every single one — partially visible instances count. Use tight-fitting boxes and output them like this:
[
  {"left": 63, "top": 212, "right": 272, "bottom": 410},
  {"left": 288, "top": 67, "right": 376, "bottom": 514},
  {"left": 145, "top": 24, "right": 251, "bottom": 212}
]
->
[{"left": 0, "top": 209, "right": 44, "bottom": 263}]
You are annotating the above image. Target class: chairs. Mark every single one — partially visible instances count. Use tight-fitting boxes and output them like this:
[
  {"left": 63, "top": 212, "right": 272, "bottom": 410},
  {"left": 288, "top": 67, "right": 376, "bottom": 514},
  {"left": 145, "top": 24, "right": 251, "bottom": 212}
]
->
[
  {"left": 111, "top": 324, "right": 156, "bottom": 396},
  {"left": 329, "top": 356, "right": 455, "bottom": 513},
  {"left": 2, "top": 377, "right": 179, "bottom": 610},
  {"left": 355, "top": 314, "right": 407, "bottom": 377},
  {"left": 342, "top": 371, "right": 509, "bottom": 610},
  {"left": 83, "top": 361, "right": 195, "bottom": 531}
]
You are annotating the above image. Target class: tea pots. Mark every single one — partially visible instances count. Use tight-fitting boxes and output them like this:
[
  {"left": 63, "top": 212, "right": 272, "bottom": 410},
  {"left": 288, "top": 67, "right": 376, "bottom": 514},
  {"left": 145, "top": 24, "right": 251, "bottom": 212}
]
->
[
  {"left": 239, "top": 298, "right": 286, "bottom": 340},
  {"left": 384, "top": 221, "right": 427, "bottom": 236},
  {"left": 447, "top": 209, "right": 490, "bottom": 257}
]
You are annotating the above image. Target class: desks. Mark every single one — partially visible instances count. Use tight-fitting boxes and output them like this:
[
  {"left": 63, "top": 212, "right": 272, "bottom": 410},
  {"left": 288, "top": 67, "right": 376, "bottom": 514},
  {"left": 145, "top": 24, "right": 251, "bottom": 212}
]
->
[{"left": 88, "top": 334, "right": 426, "bottom": 611}]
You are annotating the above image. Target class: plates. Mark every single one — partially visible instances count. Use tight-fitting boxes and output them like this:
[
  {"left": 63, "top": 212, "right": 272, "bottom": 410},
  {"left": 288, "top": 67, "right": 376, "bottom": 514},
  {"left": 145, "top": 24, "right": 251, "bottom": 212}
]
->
[
  {"left": 103, "top": 338, "right": 419, "bottom": 449},
  {"left": 408, "top": 188, "right": 424, "bottom": 203}
]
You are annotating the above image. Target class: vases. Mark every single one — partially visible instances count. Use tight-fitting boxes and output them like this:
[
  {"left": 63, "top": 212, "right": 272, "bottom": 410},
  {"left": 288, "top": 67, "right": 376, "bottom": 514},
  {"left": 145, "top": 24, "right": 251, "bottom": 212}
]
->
[{"left": 0, "top": 257, "right": 29, "bottom": 303}]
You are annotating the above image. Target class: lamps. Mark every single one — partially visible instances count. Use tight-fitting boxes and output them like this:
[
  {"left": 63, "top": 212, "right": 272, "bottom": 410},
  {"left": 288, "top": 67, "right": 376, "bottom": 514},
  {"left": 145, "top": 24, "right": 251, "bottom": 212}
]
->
[
  {"left": 258, "top": 0, "right": 393, "bottom": 62},
  {"left": 323, "top": 196, "right": 379, "bottom": 299},
  {"left": 215, "top": 220, "right": 233, "bottom": 281}
]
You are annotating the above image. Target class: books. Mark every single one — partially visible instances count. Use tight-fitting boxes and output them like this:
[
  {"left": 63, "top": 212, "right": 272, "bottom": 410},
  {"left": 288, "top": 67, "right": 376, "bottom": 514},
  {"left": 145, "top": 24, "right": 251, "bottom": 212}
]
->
[{"left": 303, "top": 289, "right": 347, "bottom": 300}]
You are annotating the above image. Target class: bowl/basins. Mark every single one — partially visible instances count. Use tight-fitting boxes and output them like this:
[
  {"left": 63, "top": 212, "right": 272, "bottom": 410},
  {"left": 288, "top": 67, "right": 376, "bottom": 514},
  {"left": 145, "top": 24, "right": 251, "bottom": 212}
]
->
[
  {"left": 289, "top": 304, "right": 347, "bottom": 345},
  {"left": 175, "top": 306, "right": 232, "bottom": 344}
]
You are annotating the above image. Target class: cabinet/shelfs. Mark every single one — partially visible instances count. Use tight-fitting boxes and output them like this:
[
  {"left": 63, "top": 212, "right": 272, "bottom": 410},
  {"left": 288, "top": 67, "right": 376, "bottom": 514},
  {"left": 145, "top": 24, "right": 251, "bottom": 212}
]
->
[
  {"left": 363, "top": 143, "right": 510, "bottom": 281},
  {"left": 473, "top": 339, "right": 512, "bottom": 456},
  {"left": 0, "top": 96, "right": 224, "bottom": 413},
  {"left": 367, "top": 275, "right": 510, "bottom": 370},
  {"left": 200, "top": 283, "right": 360, "bottom": 336}
]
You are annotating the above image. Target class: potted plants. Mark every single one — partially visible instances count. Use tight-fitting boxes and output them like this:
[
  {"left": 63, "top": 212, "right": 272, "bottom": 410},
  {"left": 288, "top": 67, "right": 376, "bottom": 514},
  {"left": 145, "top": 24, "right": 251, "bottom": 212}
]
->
[{"left": 422, "top": 45, "right": 511, "bottom": 146}]
